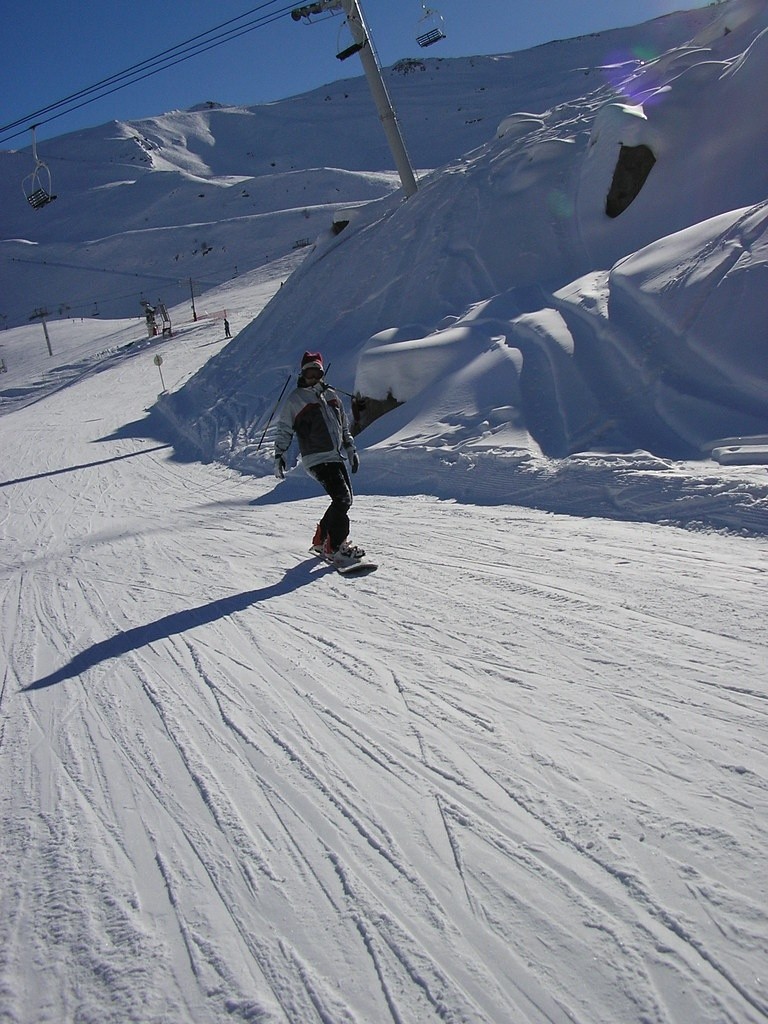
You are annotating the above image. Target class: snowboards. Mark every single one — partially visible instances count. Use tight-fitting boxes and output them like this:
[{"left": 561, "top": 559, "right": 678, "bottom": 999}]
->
[{"left": 309, "top": 542, "right": 379, "bottom": 574}]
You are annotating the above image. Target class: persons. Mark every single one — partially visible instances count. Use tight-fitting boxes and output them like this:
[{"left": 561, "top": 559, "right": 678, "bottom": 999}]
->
[
  {"left": 223, "top": 318, "right": 232, "bottom": 337},
  {"left": 272, "top": 350, "right": 365, "bottom": 563}
]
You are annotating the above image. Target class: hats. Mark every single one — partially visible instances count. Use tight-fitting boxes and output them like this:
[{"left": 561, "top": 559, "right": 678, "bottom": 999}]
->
[{"left": 301, "top": 351, "right": 325, "bottom": 375}]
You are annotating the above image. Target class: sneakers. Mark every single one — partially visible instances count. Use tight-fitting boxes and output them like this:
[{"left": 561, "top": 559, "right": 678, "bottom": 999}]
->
[
  {"left": 312, "top": 524, "right": 328, "bottom": 553},
  {"left": 324, "top": 532, "right": 365, "bottom": 561}
]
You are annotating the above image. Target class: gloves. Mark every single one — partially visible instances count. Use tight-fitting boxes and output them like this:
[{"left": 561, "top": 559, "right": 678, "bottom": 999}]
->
[
  {"left": 346, "top": 446, "right": 360, "bottom": 476},
  {"left": 273, "top": 454, "right": 287, "bottom": 480}
]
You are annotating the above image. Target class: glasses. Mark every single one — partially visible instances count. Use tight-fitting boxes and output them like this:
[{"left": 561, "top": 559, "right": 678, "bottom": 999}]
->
[{"left": 302, "top": 370, "right": 324, "bottom": 380}]
